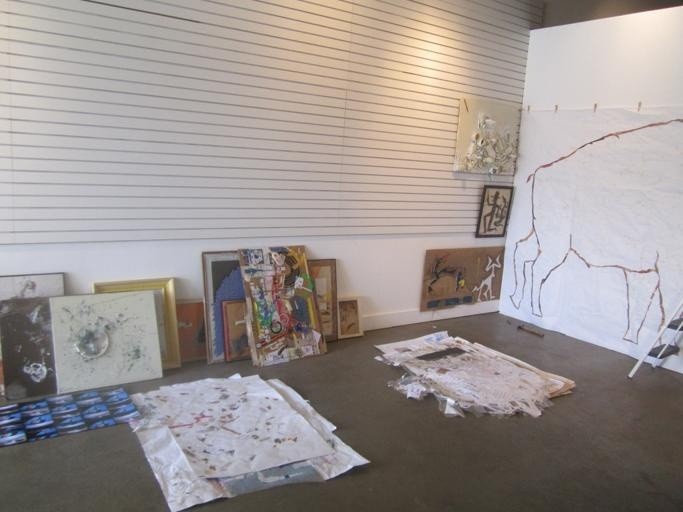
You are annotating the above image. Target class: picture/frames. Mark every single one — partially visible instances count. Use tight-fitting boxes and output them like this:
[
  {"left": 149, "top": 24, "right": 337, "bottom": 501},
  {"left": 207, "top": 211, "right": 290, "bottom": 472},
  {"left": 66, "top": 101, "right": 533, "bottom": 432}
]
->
[
  {"left": 91, "top": 250, "right": 364, "bottom": 372},
  {"left": 475, "top": 184, "right": 514, "bottom": 238}
]
